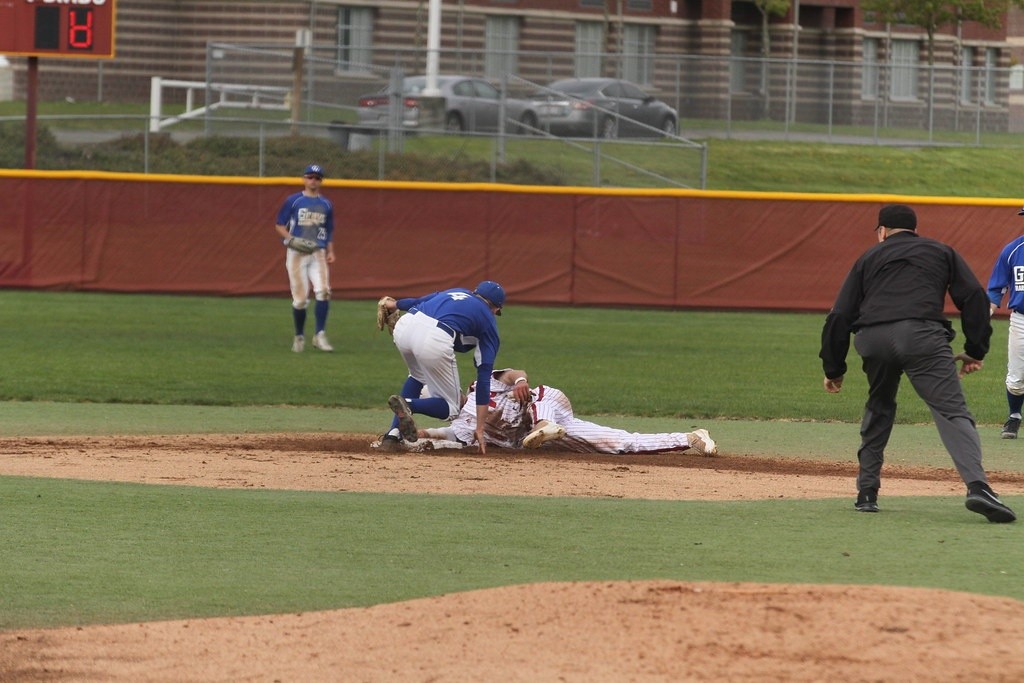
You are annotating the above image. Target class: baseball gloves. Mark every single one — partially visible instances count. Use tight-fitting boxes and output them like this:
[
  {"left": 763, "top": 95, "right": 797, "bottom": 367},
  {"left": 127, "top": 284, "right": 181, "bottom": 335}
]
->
[
  {"left": 376, "top": 295, "right": 400, "bottom": 336},
  {"left": 283, "top": 236, "right": 319, "bottom": 255}
]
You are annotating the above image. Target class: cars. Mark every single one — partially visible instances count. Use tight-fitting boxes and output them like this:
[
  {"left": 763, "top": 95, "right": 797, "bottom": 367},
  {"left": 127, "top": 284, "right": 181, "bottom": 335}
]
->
[
  {"left": 531, "top": 76, "right": 681, "bottom": 142},
  {"left": 357, "top": 74, "right": 544, "bottom": 138}
]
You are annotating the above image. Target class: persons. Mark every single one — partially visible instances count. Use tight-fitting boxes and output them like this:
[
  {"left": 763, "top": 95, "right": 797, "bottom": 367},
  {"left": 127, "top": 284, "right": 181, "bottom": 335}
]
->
[
  {"left": 986, "top": 208, "right": 1024, "bottom": 440},
  {"left": 275, "top": 164, "right": 334, "bottom": 353},
  {"left": 817, "top": 205, "right": 1017, "bottom": 522},
  {"left": 416, "top": 367, "right": 717, "bottom": 456},
  {"left": 382, "top": 282, "right": 505, "bottom": 451}
]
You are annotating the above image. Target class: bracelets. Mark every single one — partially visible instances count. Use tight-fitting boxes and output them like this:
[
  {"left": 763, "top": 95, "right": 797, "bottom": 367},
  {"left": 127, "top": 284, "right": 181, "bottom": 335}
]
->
[{"left": 514, "top": 377, "right": 528, "bottom": 385}]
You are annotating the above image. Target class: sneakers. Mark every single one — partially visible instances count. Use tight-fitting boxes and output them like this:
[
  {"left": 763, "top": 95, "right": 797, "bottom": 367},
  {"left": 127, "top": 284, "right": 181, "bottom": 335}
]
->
[
  {"left": 690, "top": 428, "right": 717, "bottom": 457},
  {"left": 522, "top": 419, "right": 565, "bottom": 448},
  {"left": 854, "top": 490, "right": 878, "bottom": 512},
  {"left": 388, "top": 394, "right": 418, "bottom": 442},
  {"left": 312, "top": 330, "right": 333, "bottom": 352},
  {"left": 965, "top": 483, "right": 1016, "bottom": 523},
  {"left": 1001, "top": 416, "right": 1021, "bottom": 439},
  {"left": 292, "top": 336, "right": 305, "bottom": 353},
  {"left": 381, "top": 434, "right": 410, "bottom": 451}
]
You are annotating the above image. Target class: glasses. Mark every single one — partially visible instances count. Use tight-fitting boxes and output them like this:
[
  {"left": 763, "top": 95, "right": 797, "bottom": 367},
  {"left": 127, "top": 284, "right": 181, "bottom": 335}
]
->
[{"left": 304, "top": 174, "right": 322, "bottom": 181}]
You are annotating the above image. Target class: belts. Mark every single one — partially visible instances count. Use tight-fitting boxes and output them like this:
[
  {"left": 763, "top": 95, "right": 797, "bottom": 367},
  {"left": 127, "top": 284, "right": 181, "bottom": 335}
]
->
[{"left": 409, "top": 307, "right": 454, "bottom": 338}]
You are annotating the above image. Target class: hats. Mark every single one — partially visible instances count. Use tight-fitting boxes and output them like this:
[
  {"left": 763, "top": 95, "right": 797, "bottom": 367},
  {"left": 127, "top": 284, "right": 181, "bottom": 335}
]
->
[
  {"left": 475, "top": 281, "right": 504, "bottom": 316},
  {"left": 303, "top": 165, "right": 324, "bottom": 178},
  {"left": 874, "top": 205, "right": 917, "bottom": 231}
]
